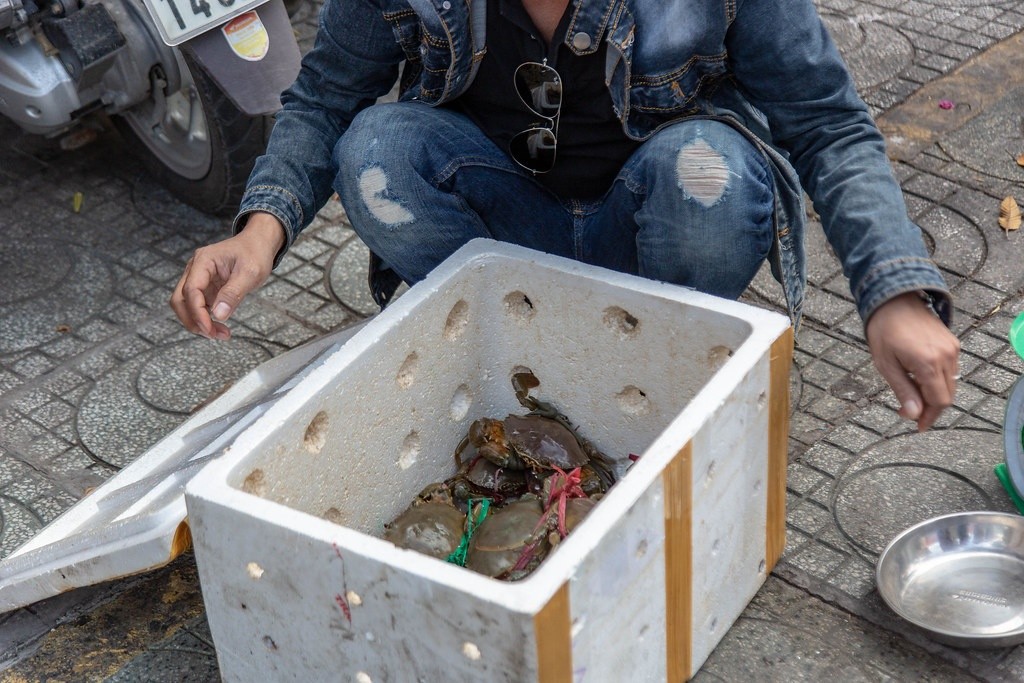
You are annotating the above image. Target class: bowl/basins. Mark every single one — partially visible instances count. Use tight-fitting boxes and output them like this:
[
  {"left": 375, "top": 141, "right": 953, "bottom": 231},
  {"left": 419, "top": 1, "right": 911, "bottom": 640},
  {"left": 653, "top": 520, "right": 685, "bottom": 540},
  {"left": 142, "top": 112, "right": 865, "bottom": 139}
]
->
[{"left": 876, "top": 511, "right": 1024, "bottom": 649}]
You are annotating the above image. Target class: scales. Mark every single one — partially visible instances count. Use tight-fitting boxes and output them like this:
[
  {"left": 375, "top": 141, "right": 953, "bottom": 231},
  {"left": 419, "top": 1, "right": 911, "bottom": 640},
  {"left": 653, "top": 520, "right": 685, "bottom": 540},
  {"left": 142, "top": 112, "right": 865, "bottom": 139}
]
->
[{"left": 993, "top": 312, "right": 1024, "bottom": 516}]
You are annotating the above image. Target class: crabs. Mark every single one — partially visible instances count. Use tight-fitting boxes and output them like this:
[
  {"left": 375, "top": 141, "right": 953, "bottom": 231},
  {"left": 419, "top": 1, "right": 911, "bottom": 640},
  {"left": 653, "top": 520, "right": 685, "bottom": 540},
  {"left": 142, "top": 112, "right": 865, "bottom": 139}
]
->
[{"left": 383, "top": 372, "right": 619, "bottom": 581}]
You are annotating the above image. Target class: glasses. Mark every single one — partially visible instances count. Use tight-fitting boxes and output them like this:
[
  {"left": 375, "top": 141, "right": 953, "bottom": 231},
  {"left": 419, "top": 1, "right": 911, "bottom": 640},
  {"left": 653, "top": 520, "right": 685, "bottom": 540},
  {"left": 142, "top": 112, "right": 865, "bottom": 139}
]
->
[{"left": 508, "top": 57, "right": 562, "bottom": 174}]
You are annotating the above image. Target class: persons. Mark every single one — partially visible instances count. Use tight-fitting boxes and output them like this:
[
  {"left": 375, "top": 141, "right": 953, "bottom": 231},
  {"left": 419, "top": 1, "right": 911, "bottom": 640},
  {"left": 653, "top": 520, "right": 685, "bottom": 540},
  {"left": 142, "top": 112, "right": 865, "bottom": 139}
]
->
[{"left": 170, "top": 0, "right": 961, "bottom": 434}]
